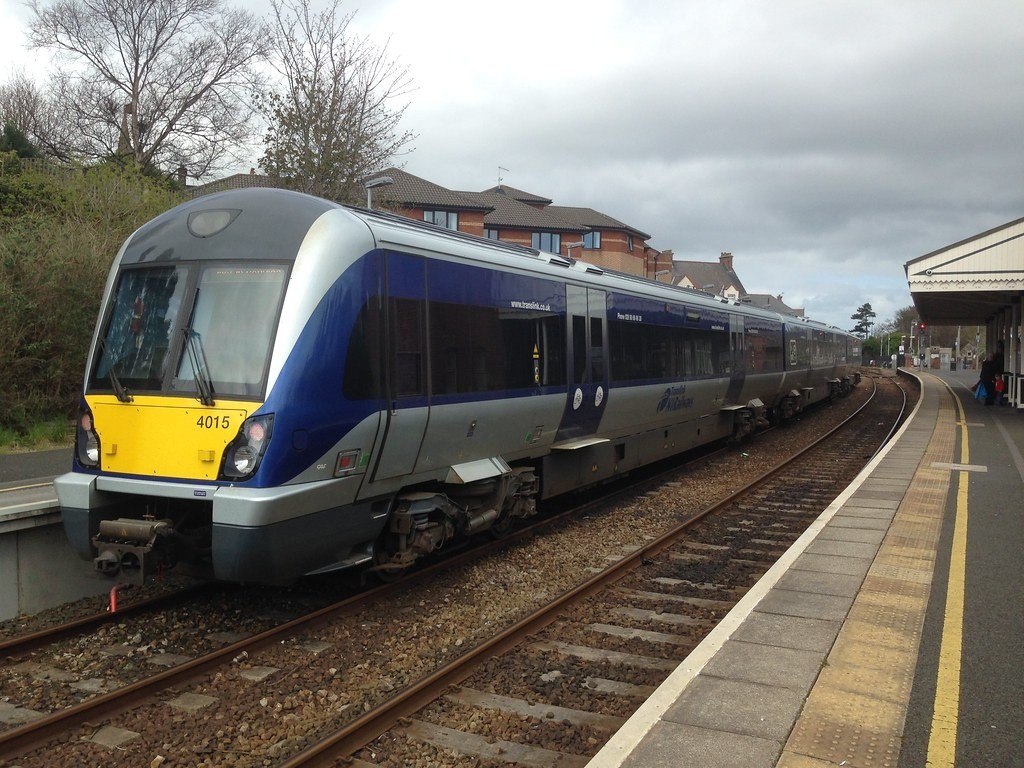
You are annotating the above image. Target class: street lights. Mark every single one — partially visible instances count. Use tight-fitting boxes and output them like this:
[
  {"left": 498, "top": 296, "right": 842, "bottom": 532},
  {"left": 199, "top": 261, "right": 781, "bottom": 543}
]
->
[
  {"left": 365, "top": 176, "right": 393, "bottom": 209},
  {"left": 564, "top": 242, "right": 585, "bottom": 258}
]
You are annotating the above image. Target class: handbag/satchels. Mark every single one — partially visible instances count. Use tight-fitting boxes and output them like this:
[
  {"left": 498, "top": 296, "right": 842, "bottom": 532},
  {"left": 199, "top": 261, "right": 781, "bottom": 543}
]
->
[{"left": 974, "top": 381, "right": 987, "bottom": 400}]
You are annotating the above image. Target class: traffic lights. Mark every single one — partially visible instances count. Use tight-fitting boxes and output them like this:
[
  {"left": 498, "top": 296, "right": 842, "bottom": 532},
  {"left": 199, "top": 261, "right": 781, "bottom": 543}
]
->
[
  {"left": 920, "top": 351, "right": 925, "bottom": 361},
  {"left": 918, "top": 321, "right": 926, "bottom": 335}
]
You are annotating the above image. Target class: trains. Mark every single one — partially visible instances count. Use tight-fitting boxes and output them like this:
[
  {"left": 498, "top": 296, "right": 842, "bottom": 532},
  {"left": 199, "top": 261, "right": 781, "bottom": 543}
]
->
[{"left": 53, "top": 188, "right": 862, "bottom": 612}]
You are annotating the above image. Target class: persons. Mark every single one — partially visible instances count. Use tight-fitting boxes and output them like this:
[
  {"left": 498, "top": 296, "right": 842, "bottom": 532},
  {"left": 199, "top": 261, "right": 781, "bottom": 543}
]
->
[
  {"left": 994, "top": 374, "right": 1005, "bottom": 405},
  {"left": 980, "top": 354, "right": 996, "bottom": 406}
]
